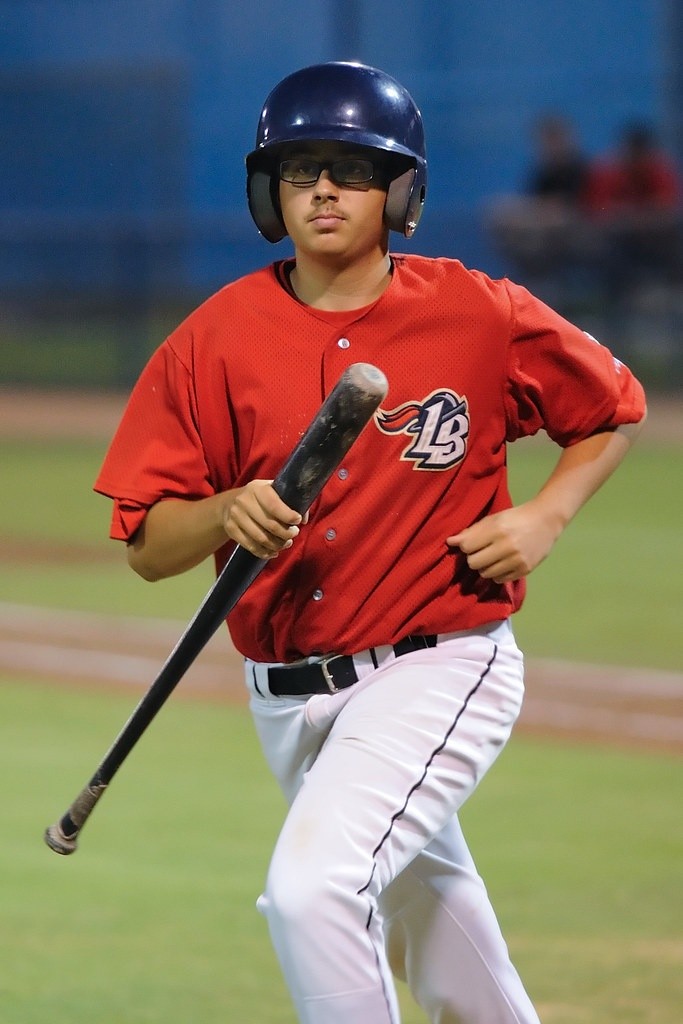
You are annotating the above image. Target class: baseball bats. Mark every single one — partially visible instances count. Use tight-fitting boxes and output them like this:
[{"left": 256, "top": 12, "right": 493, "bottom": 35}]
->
[{"left": 42, "top": 362, "right": 389, "bottom": 855}]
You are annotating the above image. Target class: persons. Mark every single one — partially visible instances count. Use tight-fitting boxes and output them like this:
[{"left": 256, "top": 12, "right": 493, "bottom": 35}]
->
[
  {"left": 92, "top": 61, "right": 647, "bottom": 1024},
  {"left": 490, "top": 112, "right": 683, "bottom": 355}
]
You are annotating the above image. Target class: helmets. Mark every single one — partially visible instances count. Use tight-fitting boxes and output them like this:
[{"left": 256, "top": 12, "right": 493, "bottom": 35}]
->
[{"left": 244, "top": 60, "right": 430, "bottom": 244}]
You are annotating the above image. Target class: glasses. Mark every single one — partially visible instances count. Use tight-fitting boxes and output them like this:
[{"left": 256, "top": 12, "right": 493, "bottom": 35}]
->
[{"left": 275, "top": 157, "right": 383, "bottom": 186}]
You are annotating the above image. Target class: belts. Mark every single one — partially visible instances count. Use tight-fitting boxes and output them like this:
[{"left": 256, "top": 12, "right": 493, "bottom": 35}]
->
[{"left": 254, "top": 632, "right": 438, "bottom": 697}]
